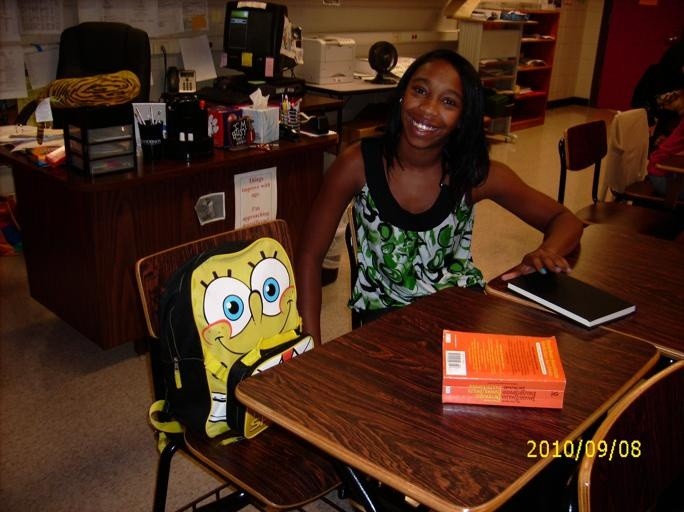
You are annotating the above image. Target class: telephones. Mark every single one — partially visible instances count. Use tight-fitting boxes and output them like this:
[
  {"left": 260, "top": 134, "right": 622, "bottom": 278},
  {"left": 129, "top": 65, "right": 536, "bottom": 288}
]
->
[{"left": 166, "top": 65, "right": 197, "bottom": 98}]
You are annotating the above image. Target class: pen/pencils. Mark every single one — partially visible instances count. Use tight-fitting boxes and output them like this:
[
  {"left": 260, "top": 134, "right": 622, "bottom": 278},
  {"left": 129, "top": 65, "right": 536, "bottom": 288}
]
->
[
  {"left": 135, "top": 105, "right": 163, "bottom": 145},
  {"left": 279, "top": 87, "right": 303, "bottom": 133}
]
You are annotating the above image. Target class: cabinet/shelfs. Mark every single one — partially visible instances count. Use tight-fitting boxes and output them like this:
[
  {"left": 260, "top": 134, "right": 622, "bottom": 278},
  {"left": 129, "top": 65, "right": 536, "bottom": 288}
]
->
[
  {"left": 455, "top": 13, "right": 519, "bottom": 135},
  {"left": 511, "top": 7, "right": 561, "bottom": 132}
]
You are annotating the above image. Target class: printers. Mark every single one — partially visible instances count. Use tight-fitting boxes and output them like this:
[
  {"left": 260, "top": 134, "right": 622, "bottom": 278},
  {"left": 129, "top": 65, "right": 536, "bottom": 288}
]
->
[{"left": 298, "top": 36, "right": 355, "bottom": 85}]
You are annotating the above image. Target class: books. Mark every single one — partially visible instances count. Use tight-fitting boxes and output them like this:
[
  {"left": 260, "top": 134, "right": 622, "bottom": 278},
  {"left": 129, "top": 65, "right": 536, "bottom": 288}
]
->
[
  {"left": 506, "top": 267, "right": 637, "bottom": 330},
  {"left": 439, "top": 327, "right": 565, "bottom": 411}
]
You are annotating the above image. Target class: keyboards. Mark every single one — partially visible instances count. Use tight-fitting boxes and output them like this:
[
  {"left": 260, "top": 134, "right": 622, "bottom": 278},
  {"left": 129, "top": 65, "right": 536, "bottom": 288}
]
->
[{"left": 196, "top": 86, "right": 249, "bottom": 106}]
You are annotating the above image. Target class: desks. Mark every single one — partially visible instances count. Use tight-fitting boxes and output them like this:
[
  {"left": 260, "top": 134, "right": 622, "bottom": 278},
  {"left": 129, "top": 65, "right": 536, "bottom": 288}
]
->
[
  {"left": 655, "top": 117, "right": 684, "bottom": 174},
  {"left": 282, "top": 89, "right": 345, "bottom": 157},
  {"left": 0, "top": 126, "right": 342, "bottom": 355},
  {"left": 306, "top": 77, "right": 405, "bottom": 153},
  {"left": 488, "top": 220, "right": 684, "bottom": 370},
  {"left": 237, "top": 285, "right": 660, "bottom": 512}
]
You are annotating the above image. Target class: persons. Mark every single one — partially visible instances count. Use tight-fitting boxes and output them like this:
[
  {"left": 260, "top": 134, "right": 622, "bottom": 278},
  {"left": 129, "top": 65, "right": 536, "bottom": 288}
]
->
[
  {"left": 631, "top": 41, "right": 684, "bottom": 193},
  {"left": 294, "top": 50, "right": 584, "bottom": 353}
]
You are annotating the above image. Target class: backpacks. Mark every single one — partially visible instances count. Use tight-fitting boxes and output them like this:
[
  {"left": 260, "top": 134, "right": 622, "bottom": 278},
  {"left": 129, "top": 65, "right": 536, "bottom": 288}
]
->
[{"left": 146, "top": 237, "right": 317, "bottom": 456}]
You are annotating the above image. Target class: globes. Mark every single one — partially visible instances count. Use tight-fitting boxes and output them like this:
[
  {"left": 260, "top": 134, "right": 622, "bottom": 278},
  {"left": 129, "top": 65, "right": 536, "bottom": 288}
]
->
[{"left": 364, "top": 40, "right": 399, "bottom": 84}]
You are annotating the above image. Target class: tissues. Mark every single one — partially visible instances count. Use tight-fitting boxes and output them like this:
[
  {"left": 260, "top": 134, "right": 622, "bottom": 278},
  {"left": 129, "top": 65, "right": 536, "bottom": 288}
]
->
[{"left": 237, "top": 87, "right": 280, "bottom": 145}]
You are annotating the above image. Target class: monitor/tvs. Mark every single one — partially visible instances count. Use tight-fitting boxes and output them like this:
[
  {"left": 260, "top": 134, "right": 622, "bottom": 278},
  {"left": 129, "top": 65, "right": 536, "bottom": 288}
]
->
[{"left": 223, "top": 1, "right": 302, "bottom": 82}]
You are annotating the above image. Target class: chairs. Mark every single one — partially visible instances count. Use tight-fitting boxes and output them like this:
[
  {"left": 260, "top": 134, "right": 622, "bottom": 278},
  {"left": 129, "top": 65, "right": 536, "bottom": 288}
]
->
[
  {"left": 13, "top": 21, "right": 153, "bottom": 136},
  {"left": 339, "top": 207, "right": 375, "bottom": 332},
  {"left": 602, "top": 107, "right": 684, "bottom": 216},
  {"left": 576, "top": 361, "right": 684, "bottom": 512},
  {"left": 555, "top": 118, "right": 674, "bottom": 243},
  {"left": 134, "top": 217, "right": 377, "bottom": 512}
]
